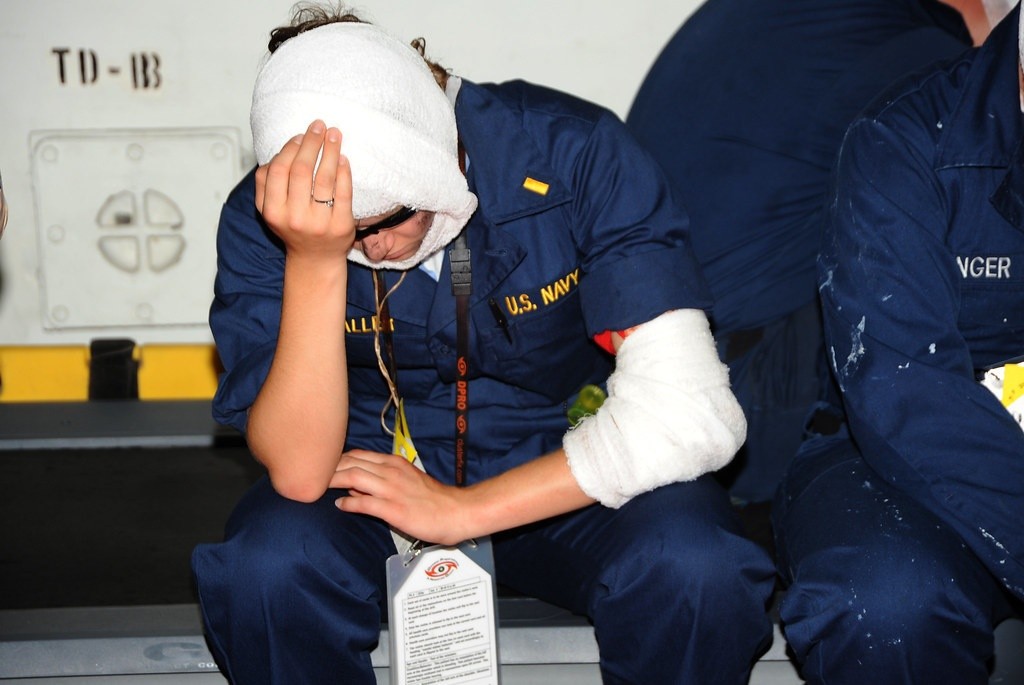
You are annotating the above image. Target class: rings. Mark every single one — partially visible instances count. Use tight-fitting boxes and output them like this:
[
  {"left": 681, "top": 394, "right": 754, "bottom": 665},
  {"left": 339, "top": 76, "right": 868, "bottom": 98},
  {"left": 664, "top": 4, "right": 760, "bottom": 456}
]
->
[{"left": 311, "top": 195, "right": 334, "bottom": 207}]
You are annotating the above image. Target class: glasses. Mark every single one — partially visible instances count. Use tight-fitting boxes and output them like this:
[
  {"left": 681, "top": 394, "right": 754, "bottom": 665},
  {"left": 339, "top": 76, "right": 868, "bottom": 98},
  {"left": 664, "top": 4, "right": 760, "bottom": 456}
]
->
[{"left": 355, "top": 206, "right": 417, "bottom": 239}]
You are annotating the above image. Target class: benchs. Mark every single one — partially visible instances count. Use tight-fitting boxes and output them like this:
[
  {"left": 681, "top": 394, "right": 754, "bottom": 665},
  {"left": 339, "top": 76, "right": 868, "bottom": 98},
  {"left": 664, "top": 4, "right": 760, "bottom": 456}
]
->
[{"left": 0, "top": 608, "right": 805, "bottom": 685}]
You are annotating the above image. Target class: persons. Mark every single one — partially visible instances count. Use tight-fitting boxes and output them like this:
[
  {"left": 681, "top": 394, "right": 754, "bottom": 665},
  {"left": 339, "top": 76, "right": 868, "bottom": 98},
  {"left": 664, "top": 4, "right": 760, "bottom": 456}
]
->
[
  {"left": 625, "top": 0, "right": 1024, "bottom": 685},
  {"left": 194, "top": 1, "right": 774, "bottom": 685}
]
